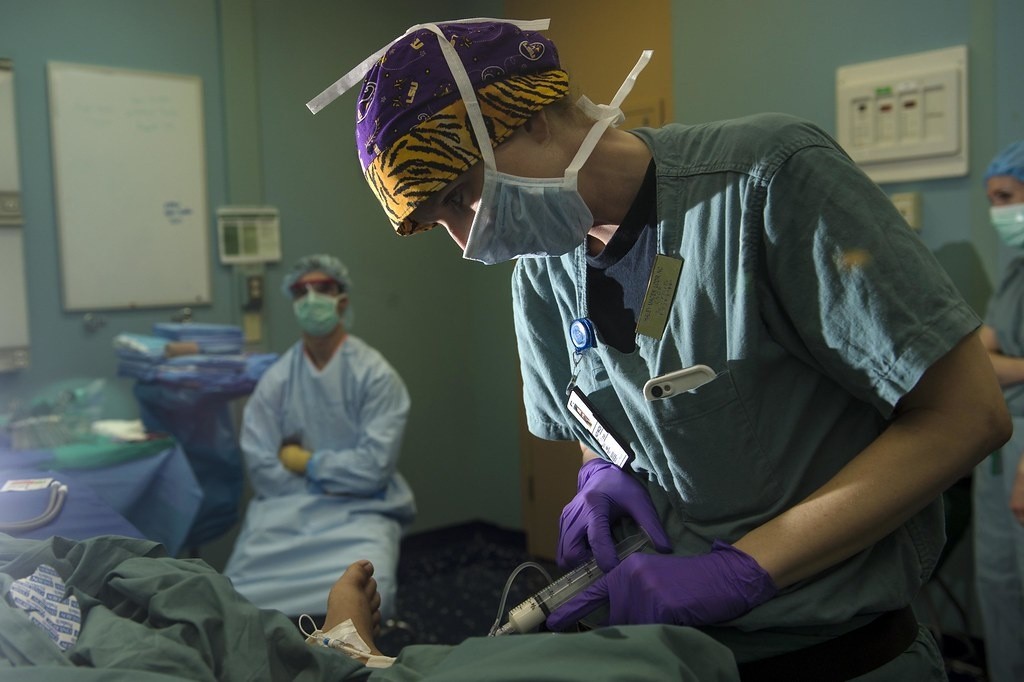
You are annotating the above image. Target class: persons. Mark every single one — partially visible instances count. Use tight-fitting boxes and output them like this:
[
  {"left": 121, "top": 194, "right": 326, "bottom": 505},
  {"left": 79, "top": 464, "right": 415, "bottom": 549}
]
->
[
  {"left": 573, "top": 405, "right": 592, "bottom": 429},
  {"left": 221, "top": 254, "right": 417, "bottom": 639},
  {"left": 303, "top": 560, "right": 388, "bottom": 669},
  {"left": 354, "top": 20, "right": 1014, "bottom": 682},
  {"left": 972, "top": 141, "right": 1024, "bottom": 682}
]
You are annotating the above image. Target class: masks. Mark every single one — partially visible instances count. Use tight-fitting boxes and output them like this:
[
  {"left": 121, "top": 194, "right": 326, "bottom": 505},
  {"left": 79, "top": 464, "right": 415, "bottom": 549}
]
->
[
  {"left": 463, "top": 166, "right": 595, "bottom": 266},
  {"left": 989, "top": 203, "right": 1024, "bottom": 250},
  {"left": 293, "top": 294, "right": 346, "bottom": 338}
]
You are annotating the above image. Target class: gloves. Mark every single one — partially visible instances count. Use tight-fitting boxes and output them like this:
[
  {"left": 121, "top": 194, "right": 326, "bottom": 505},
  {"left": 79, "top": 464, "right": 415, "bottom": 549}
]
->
[
  {"left": 277, "top": 445, "right": 311, "bottom": 475},
  {"left": 546, "top": 538, "right": 780, "bottom": 631},
  {"left": 557, "top": 457, "right": 675, "bottom": 576}
]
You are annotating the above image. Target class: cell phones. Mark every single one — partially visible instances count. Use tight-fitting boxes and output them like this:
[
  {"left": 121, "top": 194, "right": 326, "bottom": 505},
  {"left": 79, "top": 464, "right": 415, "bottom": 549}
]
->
[{"left": 643, "top": 364, "right": 717, "bottom": 401}]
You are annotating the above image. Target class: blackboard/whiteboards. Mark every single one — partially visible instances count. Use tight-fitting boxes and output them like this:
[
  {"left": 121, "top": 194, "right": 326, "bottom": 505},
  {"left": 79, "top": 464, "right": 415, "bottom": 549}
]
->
[{"left": 44, "top": 58, "right": 212, "bottom": 316}]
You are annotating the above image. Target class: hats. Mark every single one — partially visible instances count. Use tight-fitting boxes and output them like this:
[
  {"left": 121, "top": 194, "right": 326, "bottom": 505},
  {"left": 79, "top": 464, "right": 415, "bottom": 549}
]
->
[
  {"left": 983, "top": 139, "right": 1024, "bottom": 191},
  {"left": 282, "top": 254, "right": 353, "bottom": 297},
  {"left": 355, "top": 23, "right": 571, "bottom": 239}
]
